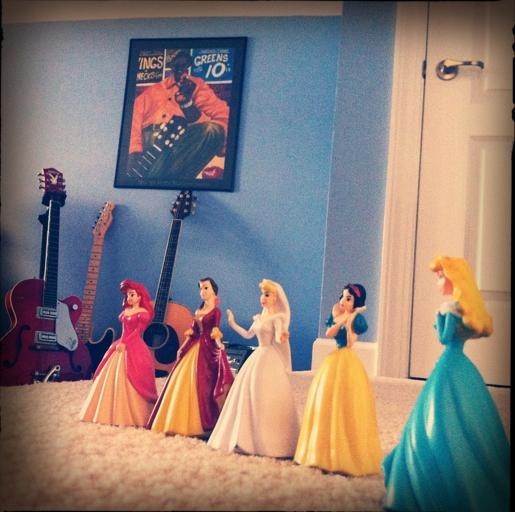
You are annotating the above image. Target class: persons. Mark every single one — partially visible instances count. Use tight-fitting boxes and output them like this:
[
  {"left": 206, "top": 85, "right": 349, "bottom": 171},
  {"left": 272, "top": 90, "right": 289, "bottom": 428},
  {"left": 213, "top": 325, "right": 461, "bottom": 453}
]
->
[
  {"left": 78, "top": 279, "right": 158, "bottom": 428},
  {"left": 126, "top": 48, "right": 230, "bottom": 180},
  {"left": 205, "top": 277, "right": 300, "bottom": 459},
  {"left": 291, "top": 282, "right": 380, "bottom": 478},
  {"left": 144, "top": 278, "right": 234, "bottom": 440},
  {"left": 378, "top": 253, "right": 512, "bottom": 512}
]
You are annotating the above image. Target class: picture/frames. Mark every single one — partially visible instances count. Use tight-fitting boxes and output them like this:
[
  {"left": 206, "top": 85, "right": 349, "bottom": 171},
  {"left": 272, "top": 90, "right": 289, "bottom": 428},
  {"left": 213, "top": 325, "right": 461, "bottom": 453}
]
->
[{"left": 113, "top": 36, "right": 249, "bottom": 194}]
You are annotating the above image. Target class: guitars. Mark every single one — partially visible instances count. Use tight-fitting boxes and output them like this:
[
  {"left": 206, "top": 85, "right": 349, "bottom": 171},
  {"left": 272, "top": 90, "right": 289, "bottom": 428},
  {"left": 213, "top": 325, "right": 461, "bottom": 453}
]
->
[
  {"left": 74, "top": 200, "right": 116, "bottom": 375},
  {"left": 142, "top": 189, "right": 201, "bottom": 377},
  {"left": 0, "top": 167, "right": 91, "bottom": 388}
]
